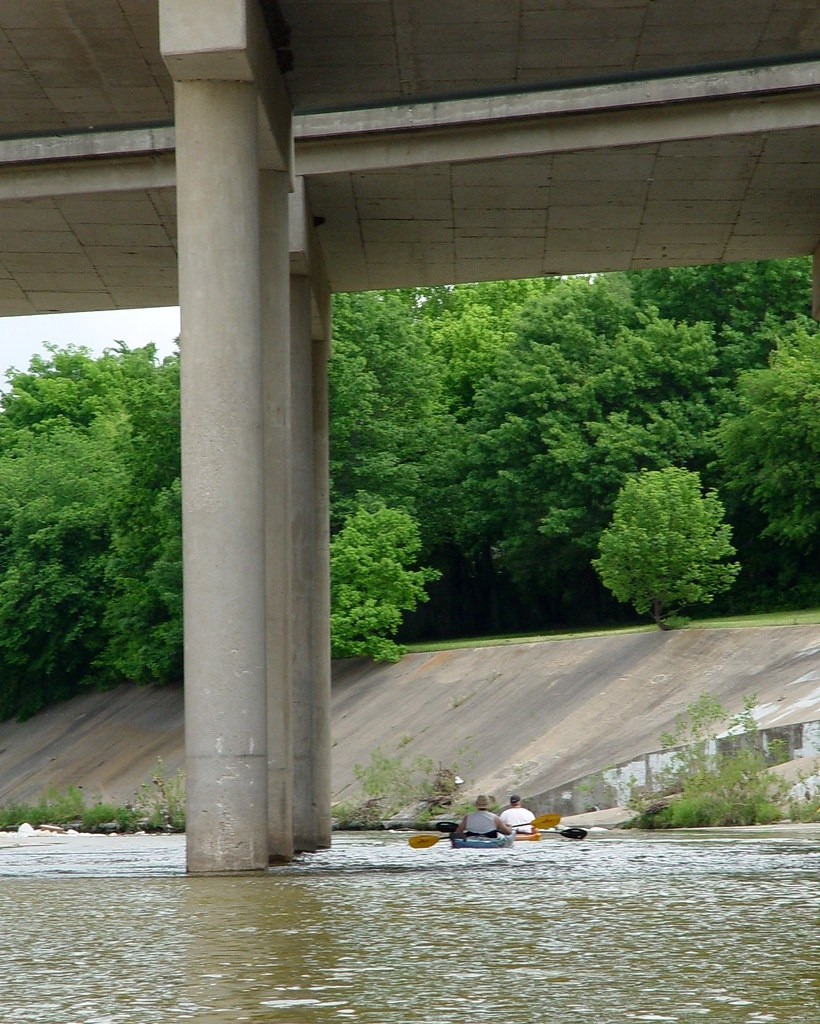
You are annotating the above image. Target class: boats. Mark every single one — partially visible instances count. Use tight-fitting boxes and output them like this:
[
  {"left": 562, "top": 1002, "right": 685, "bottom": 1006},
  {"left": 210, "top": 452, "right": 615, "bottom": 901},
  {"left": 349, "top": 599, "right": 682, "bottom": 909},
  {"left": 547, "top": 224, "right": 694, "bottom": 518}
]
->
[
  {"left": 513, "top": 832, "right": 542, "bottom": 842},
  {"left": 449, "top": 830, "right": 518, "bottom": 849}
]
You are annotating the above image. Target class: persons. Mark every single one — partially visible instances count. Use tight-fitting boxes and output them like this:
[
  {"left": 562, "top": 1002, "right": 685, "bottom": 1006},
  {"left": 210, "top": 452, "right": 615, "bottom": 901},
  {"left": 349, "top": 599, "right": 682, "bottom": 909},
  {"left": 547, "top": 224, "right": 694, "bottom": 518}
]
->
[
  {"left": 456, "top": 795, "right": 512, "bottom": 841},
  {"left": 498, "top": 795, "right": 537, "bottom": 838}
]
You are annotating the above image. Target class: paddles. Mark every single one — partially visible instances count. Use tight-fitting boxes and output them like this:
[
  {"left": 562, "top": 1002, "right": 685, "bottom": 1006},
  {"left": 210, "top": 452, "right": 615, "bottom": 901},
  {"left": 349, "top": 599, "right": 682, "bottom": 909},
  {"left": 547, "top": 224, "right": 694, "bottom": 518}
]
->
[
  {"left": 408, "top": 814, "right": 562, "bottom": 849},
  {"left": 436, "top": 822, "right": 588, "bottom": 840}
]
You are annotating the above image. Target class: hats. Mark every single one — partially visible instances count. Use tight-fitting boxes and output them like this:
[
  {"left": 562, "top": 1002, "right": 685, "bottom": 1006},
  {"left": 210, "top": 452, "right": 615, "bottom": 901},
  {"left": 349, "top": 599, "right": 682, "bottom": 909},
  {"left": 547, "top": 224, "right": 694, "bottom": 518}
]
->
[
  {"left": 474, "top": 794, "right": 496, "bottom": 809},
  {"left": 510, "top": 794, "right": 521, "bottom": 803}
]
